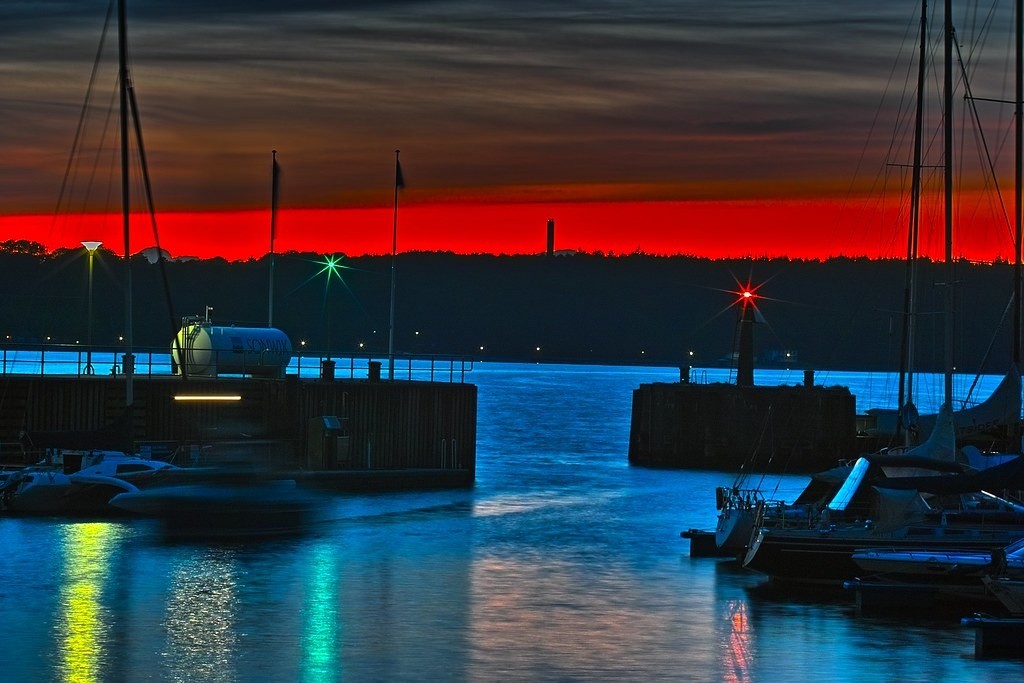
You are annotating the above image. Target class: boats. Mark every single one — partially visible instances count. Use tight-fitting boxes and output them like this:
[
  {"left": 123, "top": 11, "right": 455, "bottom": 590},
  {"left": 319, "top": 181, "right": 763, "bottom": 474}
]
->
[{"left": 1, "top": 448, "right": 315, "bottom": 536}]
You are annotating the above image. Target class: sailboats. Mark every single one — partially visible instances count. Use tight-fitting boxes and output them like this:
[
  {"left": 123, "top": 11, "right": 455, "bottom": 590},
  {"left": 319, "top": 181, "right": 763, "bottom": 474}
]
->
[{"left": 680, "top": 2, "right": 1024, "bottom": 659}]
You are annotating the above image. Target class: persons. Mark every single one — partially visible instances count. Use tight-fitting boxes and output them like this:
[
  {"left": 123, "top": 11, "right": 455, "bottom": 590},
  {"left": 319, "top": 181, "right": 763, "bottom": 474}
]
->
[
  {"left": 45, "top": 451, "right": 94, "bottom": 465},
  {"left": 820, "top": 505, "right": 831, "bottom": 529},
  {"left": 18, "top": 431, "right": 34, "bottom": 466}
]
